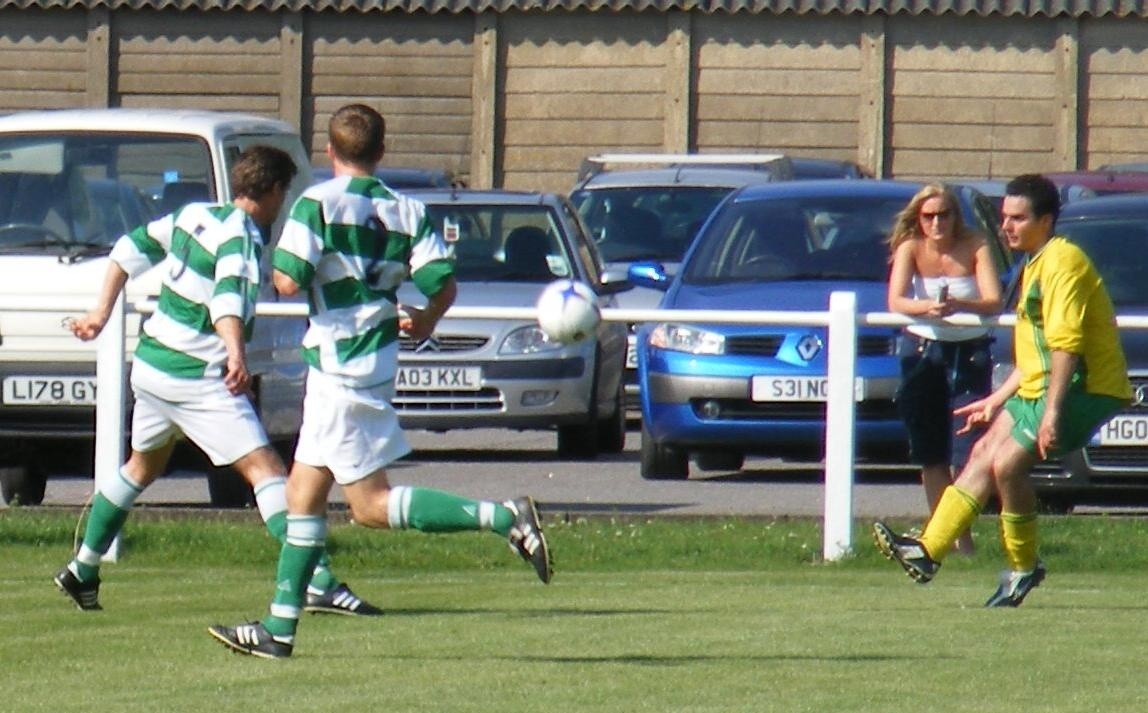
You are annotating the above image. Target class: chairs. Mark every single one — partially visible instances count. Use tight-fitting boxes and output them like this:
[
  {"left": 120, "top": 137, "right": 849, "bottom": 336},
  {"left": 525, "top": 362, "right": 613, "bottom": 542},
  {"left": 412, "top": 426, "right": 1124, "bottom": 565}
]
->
[
  {"left": 0, "top": 171, "right": 51, "bottom": 249},
  {"left": 494, "top": 225, "right": 560, "bottom": 283},
  {"left": 162, "top": 183, "right": 211, "bottom": 216},
  {"left": 602, "top": 206, "right": 662, "bottom": 260}
]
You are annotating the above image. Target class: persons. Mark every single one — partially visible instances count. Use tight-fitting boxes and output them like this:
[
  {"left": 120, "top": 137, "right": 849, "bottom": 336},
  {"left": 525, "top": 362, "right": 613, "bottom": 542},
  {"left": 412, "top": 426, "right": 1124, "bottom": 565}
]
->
[
  {"left": 203, "top": 104, "right": 551, "bottom": 660},
  {"left": 874, "top": 173, "right": 1136, "bottom": 607},
  {"left": 887, "top": 186, "right": 1007, "bottom": 553},
  {"left": 53, "top": 145, "right": 386, "bottom": 617}
]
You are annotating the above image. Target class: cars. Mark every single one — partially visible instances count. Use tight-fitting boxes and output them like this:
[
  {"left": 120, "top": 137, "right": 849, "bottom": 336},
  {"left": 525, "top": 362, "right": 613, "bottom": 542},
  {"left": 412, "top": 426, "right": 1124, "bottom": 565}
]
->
[
  {"left": 532, "top": 152, "right": 1148, "bottom": 428},
  {"left": 388, "top": 188, "right": 636, "bottom": 463},
  {"left": 980, "top": 189, "right": 1148, "bottom": 514},
  {"left": 624, "top": 176, "right": 1016, "bottom": 479},
  {"left": 311, "top": 164, "right": 495, "bottom": 265}
]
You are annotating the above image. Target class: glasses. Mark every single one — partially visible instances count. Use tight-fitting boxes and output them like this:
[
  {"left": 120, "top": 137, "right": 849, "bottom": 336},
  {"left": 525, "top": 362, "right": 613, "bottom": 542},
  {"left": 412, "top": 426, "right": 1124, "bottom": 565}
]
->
[{"left": 921, "top": 209, "right": 953, "bottom": 219}]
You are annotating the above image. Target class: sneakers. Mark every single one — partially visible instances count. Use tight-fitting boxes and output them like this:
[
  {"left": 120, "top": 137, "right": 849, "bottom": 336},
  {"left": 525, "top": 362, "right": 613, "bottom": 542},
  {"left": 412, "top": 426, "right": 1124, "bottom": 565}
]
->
[
  {"left": 207, "top": 615, "right": 294, "bottom": 661},
  {"left": 303, "top": 582, "right": 383, "bottom": 618},
  {"left": 873, "top": 522, "right": 942, "bottom": 587},
  {"left": 982, "top": 557, "right": 1046, "bottom": 607},
  {"left": 503, "top": 496, "right": 553, "bottom": 585},
  {"left": 54, "top": 561, "right": 104, "bottom": 611}
]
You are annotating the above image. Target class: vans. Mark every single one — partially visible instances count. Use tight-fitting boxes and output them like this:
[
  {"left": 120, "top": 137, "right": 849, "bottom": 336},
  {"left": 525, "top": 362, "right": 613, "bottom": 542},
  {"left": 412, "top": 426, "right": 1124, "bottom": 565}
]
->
[{"left": 1, "top": 103, "right": 321, "bottom": 509}]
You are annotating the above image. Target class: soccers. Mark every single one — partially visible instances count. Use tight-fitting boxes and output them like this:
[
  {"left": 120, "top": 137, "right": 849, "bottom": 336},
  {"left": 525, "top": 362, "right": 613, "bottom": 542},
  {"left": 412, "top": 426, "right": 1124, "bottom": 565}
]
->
[{"left": 536, "top": 278, "right": 602, "bottom": 346}]
